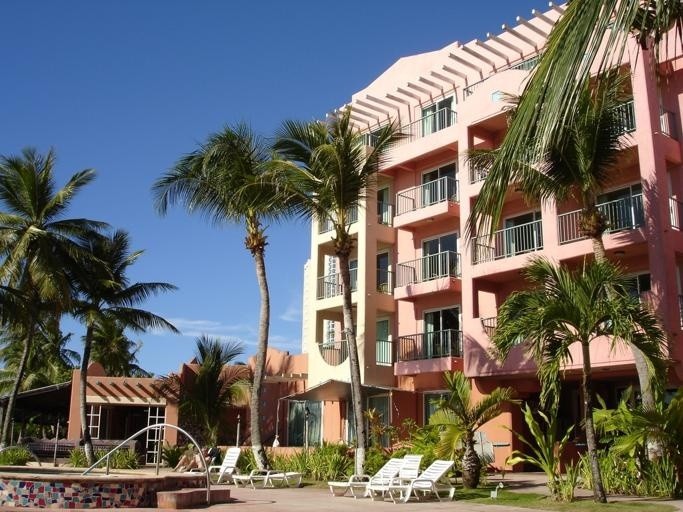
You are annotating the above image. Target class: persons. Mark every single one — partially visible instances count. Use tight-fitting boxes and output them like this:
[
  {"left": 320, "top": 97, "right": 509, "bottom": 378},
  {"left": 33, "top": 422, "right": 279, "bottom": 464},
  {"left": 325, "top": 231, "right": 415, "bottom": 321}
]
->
[
  {"left": 194, "top": 447, "right": 210, "bottom": 471},
  {"left": 172, "top": 443, "right": 198, "bottom": 472}
]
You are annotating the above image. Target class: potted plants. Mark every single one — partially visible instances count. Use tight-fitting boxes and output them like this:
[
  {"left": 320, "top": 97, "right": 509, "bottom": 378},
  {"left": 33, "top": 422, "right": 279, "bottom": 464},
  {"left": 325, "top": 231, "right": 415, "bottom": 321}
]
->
[{"left": 431, "top": 369, "right": 524, "bottom": 490}]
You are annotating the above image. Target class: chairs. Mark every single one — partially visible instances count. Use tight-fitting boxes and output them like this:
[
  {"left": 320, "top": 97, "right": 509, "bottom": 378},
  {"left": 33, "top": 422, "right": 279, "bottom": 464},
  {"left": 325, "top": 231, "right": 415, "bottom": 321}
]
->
[
  {"left": 326, "top": 454, "right": 456, "bottom": 504},
  {"left": 182, "top": 447, "right": 305, "bottom": 490}
]
